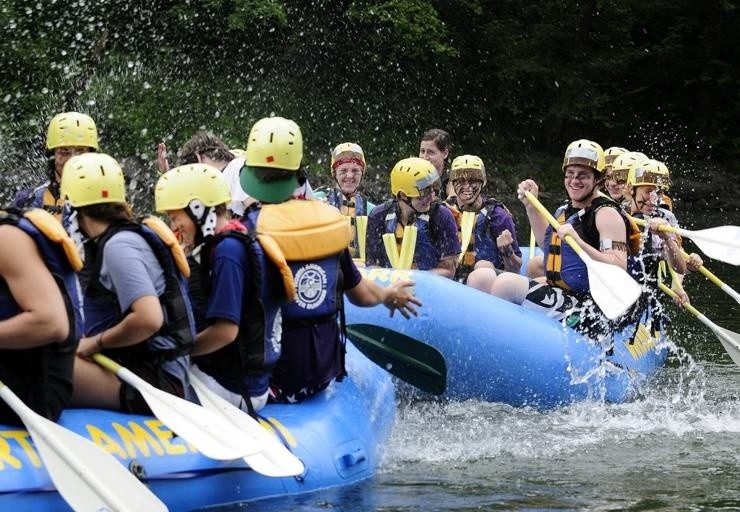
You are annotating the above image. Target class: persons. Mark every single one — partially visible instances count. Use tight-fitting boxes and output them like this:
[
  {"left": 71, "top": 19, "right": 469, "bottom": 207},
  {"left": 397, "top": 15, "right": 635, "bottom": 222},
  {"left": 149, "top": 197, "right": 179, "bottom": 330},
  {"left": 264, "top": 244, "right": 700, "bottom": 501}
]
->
[
  {"left": 1, "top": 112, "right": 422, "bottom": 428},
  {"left": 313, "top": 130, "right": 704, "bottom": 340}
]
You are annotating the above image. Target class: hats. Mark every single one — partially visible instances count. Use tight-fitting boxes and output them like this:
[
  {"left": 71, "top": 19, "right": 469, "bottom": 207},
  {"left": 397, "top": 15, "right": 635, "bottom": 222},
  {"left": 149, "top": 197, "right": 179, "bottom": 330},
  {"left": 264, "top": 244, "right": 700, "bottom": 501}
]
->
[
  {"left": 239, "top": 165, "right": 299, "bottom": 204},
  {"left": 563, "top": 137, "right": 606, "bottom": 173}
]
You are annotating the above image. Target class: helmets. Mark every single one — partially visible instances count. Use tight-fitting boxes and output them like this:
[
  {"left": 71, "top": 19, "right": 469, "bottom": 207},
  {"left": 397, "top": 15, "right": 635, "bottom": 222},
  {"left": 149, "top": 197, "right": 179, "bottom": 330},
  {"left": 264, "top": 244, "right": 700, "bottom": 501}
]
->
[
  {"left": 154, "top": 164, "right": 232, "bottom": 213},
  {"left": 244, "top": 116, "right": 305, "bottom": 171},
  {"left": 448, "top": 154, "right": 486, "bottom": 184},
  {"left": 331, "top": 141, "right": 367, "bottom": 177},
  {"left": 58, "top": 152, "right": 127, "bottom": 209},
  {"left": 389, "top": 156, "right": 440, "bottom": 198},
  {"left": 46, "top": 111, "right": 98, "bottom": 149},
  {"left": 604, "top": 147, "right": 671, "bottom": 188}
]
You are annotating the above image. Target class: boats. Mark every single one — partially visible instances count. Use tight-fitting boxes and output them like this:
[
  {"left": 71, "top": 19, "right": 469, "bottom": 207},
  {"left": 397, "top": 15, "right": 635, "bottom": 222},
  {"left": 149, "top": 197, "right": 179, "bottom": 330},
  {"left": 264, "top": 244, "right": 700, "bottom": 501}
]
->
[
  {"left": 342, "top": 248, "right": 669, "bottom": 414},
  {"left": 0, "top": 335, "right": 397, "bottom": 512}
]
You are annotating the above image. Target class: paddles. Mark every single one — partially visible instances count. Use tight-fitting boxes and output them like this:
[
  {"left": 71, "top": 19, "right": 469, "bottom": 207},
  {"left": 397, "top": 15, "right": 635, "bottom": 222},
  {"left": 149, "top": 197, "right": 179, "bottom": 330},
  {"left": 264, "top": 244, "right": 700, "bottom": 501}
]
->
[
  {"left": 188, "top": 371, "right": 303, "bottom": 478},
  {"left": 517, "top": 188, "right": 643, "bottom": 319},
  {"left": 340, "top": 323, "right": 446, "bottom": 395},
  {"left": 0, "top": 381, "right": 171, "bottom": 511},
  {"left": 91, "top": 351, "right": 257, "bottom": 459},
  {"left": 630, "top": 216, "right": 740, "bottom": 268},
  {"left": 655, "top": 282, "right": 740, "bottom": 367}
]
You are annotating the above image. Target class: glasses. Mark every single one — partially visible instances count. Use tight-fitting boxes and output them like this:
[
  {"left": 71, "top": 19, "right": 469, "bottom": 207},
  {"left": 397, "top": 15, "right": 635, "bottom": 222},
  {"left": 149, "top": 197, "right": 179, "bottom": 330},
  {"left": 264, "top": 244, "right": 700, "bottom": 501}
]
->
[
  {"left": 58, "top": 148, "right": 85, "bottom": 156},
  {"left": 419, "top": 184, "right": 440, "bottom": 197}
]
